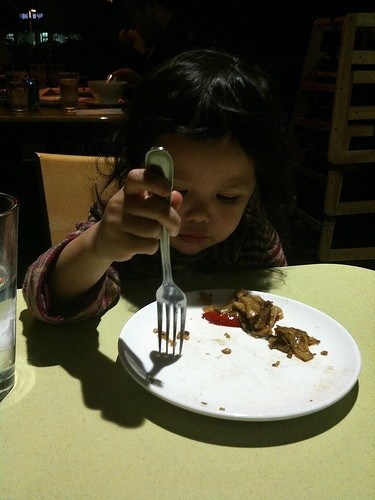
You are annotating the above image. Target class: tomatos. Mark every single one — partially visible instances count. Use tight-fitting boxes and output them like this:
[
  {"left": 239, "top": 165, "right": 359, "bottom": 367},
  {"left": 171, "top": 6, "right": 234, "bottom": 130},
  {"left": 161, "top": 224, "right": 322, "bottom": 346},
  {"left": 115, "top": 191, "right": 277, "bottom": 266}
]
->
[{"left": 203, "top": 310, "right": 240, "bottom": 326}]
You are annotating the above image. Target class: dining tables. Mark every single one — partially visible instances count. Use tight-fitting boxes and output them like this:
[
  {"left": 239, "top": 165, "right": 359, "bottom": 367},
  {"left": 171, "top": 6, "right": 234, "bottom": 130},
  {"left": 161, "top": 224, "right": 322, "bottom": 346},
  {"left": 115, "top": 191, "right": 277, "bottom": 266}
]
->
[
  {"left": 0, "top": 264, "right": 375, "bottom": 500},
  {"left": 0, "top": 88, "right": 130, "bottom": 152}
]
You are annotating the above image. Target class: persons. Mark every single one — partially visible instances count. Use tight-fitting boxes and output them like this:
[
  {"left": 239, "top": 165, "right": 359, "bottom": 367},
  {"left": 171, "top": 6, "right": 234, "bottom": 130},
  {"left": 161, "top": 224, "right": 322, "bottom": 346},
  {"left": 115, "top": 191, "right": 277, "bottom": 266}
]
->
[
  {"left": 91, "top": 0, "right": 184, "bottom": 80},
  {"left": 22, "top": 49, "right": 289, "bottom": 326}
]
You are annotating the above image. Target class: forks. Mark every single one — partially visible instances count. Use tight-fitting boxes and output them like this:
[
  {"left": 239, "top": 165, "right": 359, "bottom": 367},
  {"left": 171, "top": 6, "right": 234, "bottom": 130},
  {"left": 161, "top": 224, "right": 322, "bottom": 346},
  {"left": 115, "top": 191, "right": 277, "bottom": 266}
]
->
[{"left": 146, "top": 147, "right": 186, "bottom": 355}]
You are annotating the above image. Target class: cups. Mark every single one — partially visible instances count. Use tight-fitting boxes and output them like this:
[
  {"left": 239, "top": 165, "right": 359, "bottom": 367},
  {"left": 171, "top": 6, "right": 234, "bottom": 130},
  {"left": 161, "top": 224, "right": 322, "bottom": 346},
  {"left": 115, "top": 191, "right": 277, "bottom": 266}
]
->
[
  {"left": 57, "top": 71, "right": 78, "bottom": 113},
  {"left": 0, "top": 192, "right": 20, "bottom": 400},
  {"left": 4, "top": 72, "right": 31, "bottom": 112}
]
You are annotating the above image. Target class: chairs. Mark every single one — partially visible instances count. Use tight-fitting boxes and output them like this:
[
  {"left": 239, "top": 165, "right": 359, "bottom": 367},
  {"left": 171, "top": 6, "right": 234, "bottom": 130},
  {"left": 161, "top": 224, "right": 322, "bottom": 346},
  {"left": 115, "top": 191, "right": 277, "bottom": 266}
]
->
[{"left": 33, "top": 153, "right": 122, "bottom": 250}]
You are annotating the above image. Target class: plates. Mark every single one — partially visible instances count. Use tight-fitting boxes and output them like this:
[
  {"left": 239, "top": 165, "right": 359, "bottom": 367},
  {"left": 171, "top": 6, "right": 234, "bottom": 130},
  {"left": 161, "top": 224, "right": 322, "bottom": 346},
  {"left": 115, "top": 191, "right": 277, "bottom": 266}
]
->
[
  {"left": 118, "top": 288, "right": 362, "bottom": 422},
  {"left": 86, "top": 98, "right": 126, "bottom": 107}
]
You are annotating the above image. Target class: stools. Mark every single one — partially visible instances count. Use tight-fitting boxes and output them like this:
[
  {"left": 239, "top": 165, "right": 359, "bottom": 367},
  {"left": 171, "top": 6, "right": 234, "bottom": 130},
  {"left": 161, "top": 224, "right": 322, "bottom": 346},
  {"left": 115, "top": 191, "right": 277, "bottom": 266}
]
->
[{"left": 285, "top": 13, "right": 375, "bottom": 261}]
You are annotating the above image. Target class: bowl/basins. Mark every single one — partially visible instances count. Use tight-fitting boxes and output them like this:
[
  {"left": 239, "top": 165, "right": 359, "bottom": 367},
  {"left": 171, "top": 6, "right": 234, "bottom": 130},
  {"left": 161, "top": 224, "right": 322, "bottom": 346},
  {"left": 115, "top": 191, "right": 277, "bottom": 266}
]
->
[{"left": 87, "top": 81, "right": 129, "bottom": 104}]
